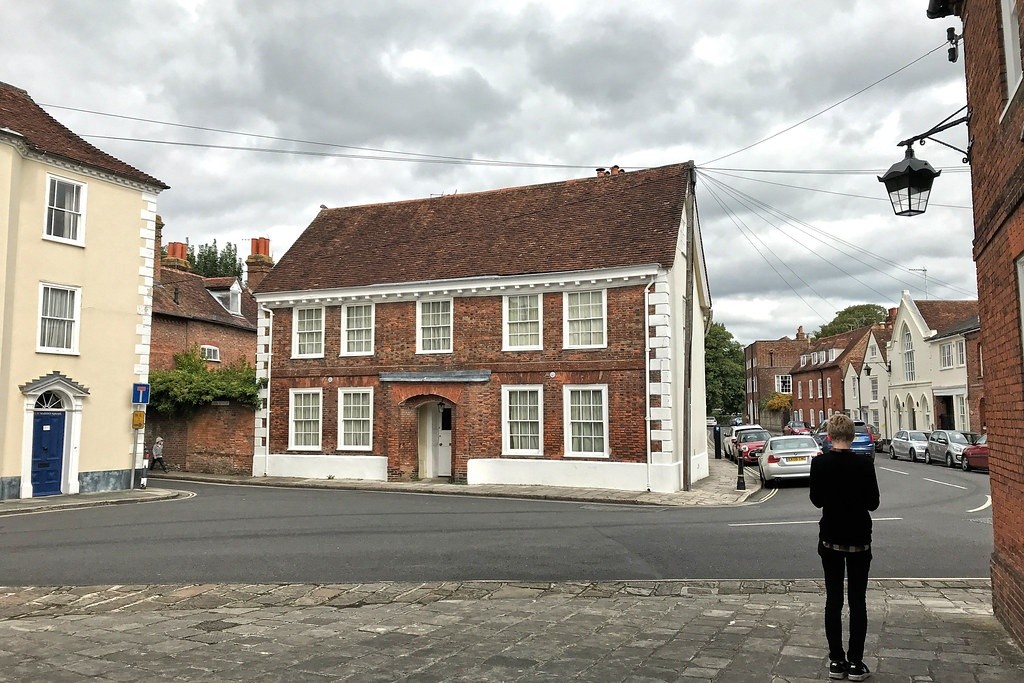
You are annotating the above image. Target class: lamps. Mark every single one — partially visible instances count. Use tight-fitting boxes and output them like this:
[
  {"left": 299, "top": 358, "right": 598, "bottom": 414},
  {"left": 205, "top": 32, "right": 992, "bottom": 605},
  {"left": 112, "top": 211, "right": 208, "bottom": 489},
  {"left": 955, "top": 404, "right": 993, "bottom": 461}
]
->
[
  {"left": 864, "top": 360, "right": 891, "bottom": 376},
  {"left": 876, "top": 102, "right": 973, "bottom": 216}
]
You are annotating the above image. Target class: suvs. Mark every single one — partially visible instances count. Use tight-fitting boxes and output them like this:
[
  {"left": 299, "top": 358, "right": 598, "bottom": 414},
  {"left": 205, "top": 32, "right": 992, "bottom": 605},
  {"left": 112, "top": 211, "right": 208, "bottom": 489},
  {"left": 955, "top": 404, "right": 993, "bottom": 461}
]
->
[{"left": 813, "top": 419, "right": 875, "bottom": 464}]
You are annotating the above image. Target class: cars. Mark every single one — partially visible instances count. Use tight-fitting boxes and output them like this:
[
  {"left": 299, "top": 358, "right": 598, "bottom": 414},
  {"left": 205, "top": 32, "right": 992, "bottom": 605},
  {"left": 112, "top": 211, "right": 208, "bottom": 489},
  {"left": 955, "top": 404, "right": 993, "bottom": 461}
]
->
[
  {"left": 754, "top": 435, "right": 824, "bottom": 487},
  {"left": 961, "top": 433, "right": 990, "bottom": 471},
  {"left": 722, "top": 424, "right": 766, "bottom": 461},
  {"left": 731, "top": 430, "right": 780, "bottom": 466},
  {"left": 866, "top": 424, "right": 883, "bottom": 453},
  {"left": 706, "top": 417, "right": 718, "bottom": 428},
  {"left": 783, "top": 421, "right": 814, "bottom": 436},
  {"left": 925, "top": 429, "right": 980, "bottom": 468},
  {"left": 888, "top": 430, "right": 934, "bottom": 462}
]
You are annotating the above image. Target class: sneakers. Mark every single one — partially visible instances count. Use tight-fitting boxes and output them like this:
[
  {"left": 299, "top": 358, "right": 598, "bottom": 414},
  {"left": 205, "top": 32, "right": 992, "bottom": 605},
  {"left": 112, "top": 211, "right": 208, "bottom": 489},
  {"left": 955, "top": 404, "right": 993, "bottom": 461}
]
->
[{"left": 829, "top": 661, "right": 871, "bottom": 680}]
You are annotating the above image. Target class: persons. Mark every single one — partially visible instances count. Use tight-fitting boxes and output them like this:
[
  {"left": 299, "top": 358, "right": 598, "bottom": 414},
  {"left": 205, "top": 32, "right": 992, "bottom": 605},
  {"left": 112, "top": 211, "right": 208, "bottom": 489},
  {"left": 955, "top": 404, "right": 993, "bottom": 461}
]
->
[
  {"left": 149, "top": 437, "right": 170, "bottom": 473},
  {"left": 810, "top": 413, "right": 881, "bottom": 680}
]
